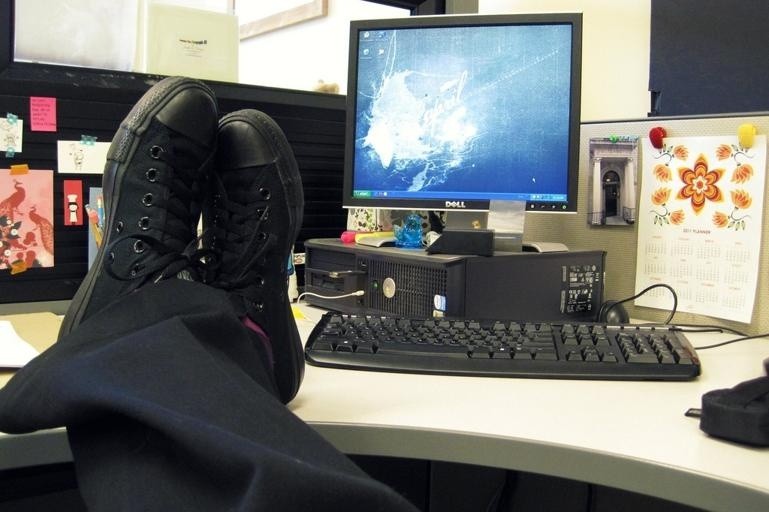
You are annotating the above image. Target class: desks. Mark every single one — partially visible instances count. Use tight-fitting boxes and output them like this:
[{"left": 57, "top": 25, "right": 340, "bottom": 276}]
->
[{"left": 0, "top": 299, "right": 769, "bottom": 512}]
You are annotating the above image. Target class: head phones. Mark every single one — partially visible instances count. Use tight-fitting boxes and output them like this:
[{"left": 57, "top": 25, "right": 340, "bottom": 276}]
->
[{"left": 597, "top": 284, "right": 677, "bottom": 324}]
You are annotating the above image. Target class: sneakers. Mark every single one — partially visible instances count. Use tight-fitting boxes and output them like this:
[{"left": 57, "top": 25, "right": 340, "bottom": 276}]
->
[
  {"left": 202, "top": 108, "right": 305, "bottom": 404},
  {"left": 56, "top": 75, "right": 221, "bottom": 343}
]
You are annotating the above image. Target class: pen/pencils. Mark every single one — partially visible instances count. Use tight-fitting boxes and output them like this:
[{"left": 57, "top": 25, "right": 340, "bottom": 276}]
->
[
  {"left": 84, "top": 195, "right": 106, "bottom": 249},
  {"left": 340, "top": 231, "right": 394, "bottom": 244}
]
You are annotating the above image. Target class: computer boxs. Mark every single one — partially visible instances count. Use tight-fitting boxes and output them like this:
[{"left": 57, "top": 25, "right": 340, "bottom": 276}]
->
[{"left": 304, "top": 235, "right": 605, "bottom": 323}]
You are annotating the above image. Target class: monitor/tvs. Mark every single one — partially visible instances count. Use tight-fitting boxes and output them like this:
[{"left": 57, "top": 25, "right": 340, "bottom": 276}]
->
[{"left": 342, "top": 13, "right": 583, "bottom": 253}]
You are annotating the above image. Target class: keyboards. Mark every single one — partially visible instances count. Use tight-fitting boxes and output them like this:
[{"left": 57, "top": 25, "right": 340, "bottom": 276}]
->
[{"left": 304, "top": 312, "right": 700, "bottom": 382}]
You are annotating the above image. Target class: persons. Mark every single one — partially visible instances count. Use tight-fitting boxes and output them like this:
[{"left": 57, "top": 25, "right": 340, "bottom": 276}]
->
[{"left": 2, "top": 75, "right": 422, "bottom": 511}]
